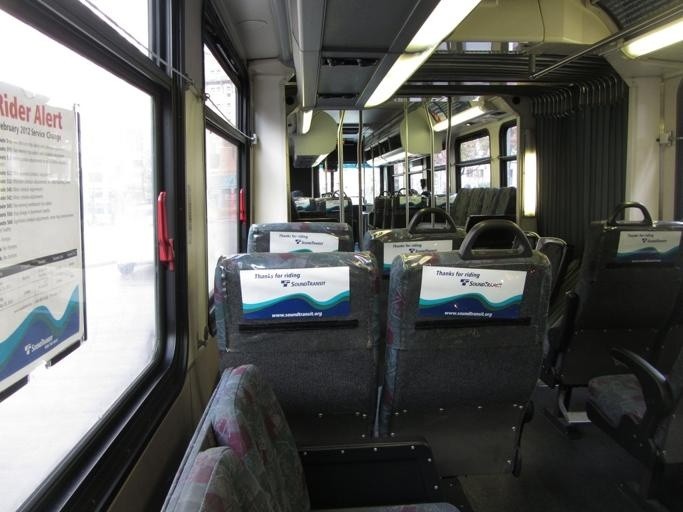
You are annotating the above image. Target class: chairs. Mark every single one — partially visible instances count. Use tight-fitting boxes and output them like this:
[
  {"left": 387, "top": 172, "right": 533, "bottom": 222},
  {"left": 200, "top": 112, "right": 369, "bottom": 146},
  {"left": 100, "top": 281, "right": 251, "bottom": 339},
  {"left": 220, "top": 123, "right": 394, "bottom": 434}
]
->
[
  {"left": 158, "top": 222, "right": 552, "bottom": 512},
  {"left": 292, "top": 187, "right": 517, "bottom": 222},
  {"left": 552, "top": 202, "right": 683, "bottom": 512}
]
